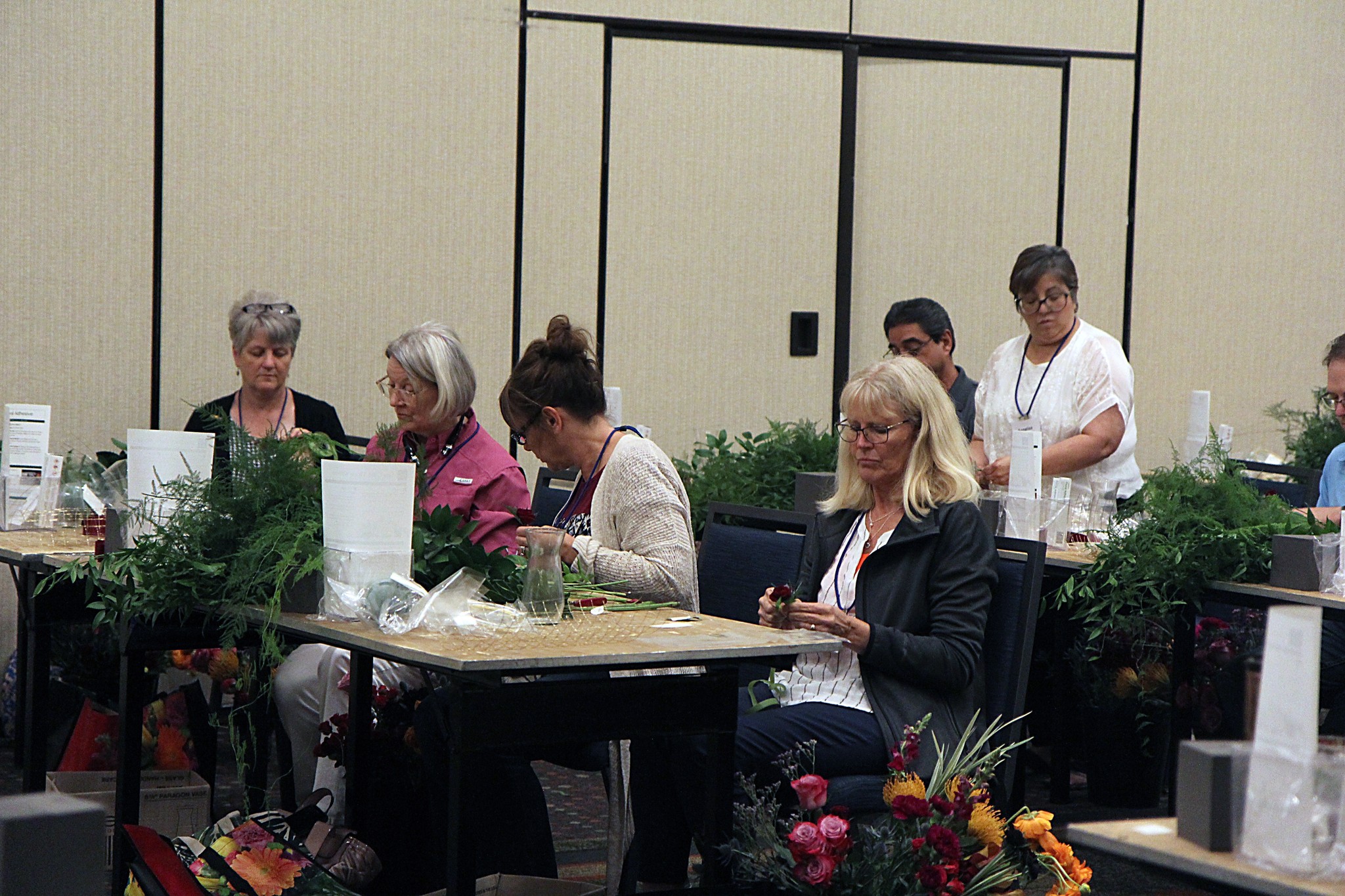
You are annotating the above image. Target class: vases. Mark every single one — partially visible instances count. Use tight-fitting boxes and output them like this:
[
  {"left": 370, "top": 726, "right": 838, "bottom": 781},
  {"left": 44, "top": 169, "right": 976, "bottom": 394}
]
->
[
  {"left": 1089, "top": 480, "right": 1120, "bottom": 544},
  {"left": 523, "top": 528, "right": 566, "bottom": 624}
]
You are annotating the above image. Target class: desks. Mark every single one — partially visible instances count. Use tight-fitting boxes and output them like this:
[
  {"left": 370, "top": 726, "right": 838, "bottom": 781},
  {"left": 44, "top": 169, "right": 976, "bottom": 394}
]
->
[
  {"left": 776, "top": 529, "right": 1345, "bottom": 814},
  {"left": 45, "top": 555, "right": 853, "bottom": 896},
  {"left": 0, "top": 527, "right": 105, "bottom": 769},
  {"left": 1068, "top": 818, "right": 1344, "bottom": 896}
]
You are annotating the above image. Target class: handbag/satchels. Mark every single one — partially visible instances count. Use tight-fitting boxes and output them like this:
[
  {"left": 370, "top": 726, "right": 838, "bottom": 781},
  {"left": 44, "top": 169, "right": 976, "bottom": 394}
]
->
[
  {"left": 56, "top": 690, "right": 190, "bottom": 770},
  {"left": 122, "top": 784, "right": 380, "bottom": 896},
  {"left": 427, "top": 870, "right": 611, "bottom": 895}
]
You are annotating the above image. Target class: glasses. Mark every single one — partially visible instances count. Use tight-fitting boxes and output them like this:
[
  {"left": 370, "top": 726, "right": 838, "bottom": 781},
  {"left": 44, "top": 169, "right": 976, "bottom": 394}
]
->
[
  {"left": 375, "top": 376, "right": 423, "bottom": 405},
  {"left": 836, "top": 418, "right": 909, "bottom": 444},
  {"left": 884, "top": 339, "right": 931, "bottom": 358},
  {"left": 1319, "top": 392, "right": 1345, "bottom": 412},
  {"left": 1014, "top": 290, "right": 1073, "bottom": 316},
  {"left": 239, "top": 304, "right": 295, "bottom": 315},
  {"left": 511, "top": 410, "right": 541, "bottom": 445}
]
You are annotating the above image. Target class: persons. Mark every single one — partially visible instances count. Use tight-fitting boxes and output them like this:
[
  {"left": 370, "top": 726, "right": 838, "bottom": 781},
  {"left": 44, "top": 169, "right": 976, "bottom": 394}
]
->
[
  {"left": 273, "top": 324, "right": 532, "bottom": 831},
  {"left": 1196, "top": 333, "right": 1345, "bottom": 741},
  {"left": 411, "top": 314, "right": 702, "bottom": 879},
  {"left": 964, "top": 244, "right": 1149, "bottom": 790},
  {"left": 179, "top": 290, "right": 352, "bottom": 814},
  {"left": 695, "top": 357, "right": 997, "bottom": 896},
  {"left": 883, "top": 296, "right": 981, "bottom": 450}
]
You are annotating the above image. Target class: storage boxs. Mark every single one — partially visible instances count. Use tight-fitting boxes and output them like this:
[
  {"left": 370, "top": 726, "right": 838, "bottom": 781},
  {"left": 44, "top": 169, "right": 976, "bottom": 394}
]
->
[
  {"left": 322, "top": 548, "right": 415, "bottom": 621},
  {"left": 46, "top": 771, "right": 212, "bottom": 871},
  {"left": 1231, "top": 748, "right": 1345, "bottom": 884},
  {"left": 421, "top": 872, "right": 607, "bottom": 896},
  {"left": 986, "top": 496, "right": 1071, "bottom": 552},
  {"left": 0, "top": 475, "right": 83, "bottom": 535}
]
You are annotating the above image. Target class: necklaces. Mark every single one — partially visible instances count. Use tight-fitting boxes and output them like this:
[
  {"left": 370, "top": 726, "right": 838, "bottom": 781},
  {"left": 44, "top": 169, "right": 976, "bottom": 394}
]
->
[{"left": 863, "top": 496, "right": 904, "bottom": 549}]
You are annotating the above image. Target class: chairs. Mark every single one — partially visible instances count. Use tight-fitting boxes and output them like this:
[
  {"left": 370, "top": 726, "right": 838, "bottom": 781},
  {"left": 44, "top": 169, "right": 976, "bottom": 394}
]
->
[{"left": 343, "top": 436, "right": 1323, "bottom": 896}]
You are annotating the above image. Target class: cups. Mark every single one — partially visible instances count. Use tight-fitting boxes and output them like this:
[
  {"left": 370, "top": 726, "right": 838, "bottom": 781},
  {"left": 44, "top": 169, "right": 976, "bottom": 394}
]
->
[
  {"left": 1088, "top": 479, "right": 1120, "bottom": 542},
  {"left": 1230, "top": 737, "right": 1343, "bottom": 880},
  {"left": 1322, "top": 534, "right": 1344, "bottom": 588},
  {"left": 523, "top": 527, "right": 564, "bottom": 625}
]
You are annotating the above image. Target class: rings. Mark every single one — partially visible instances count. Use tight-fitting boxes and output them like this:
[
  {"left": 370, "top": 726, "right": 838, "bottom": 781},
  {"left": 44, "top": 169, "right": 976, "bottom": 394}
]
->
[{"left": 810, "top": 623, "right": 816, "bottom": 630}]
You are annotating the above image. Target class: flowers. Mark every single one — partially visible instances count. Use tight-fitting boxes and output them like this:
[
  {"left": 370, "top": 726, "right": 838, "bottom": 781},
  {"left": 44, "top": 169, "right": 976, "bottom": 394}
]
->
[
  {"left": 162, "top": 648, "right": 280, "bottom": 821},
  {"left": 682, "top": 708, "right": 1092, "bottom": 896},
  {"left": 1030, "top": 384, "right": 1344, "bottom": 755},
  {"left": 746, "top": 580, "right": 806, "bottom": 714},
  {"left": 312, "top": 670, "right": 443, "bottom": 780}
]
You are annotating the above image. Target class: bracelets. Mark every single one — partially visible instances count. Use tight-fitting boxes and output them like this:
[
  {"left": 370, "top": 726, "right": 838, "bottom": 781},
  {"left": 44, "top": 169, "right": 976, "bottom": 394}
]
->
[{"left": 1339, "top": 505, "right": 1345, "bottom": 527}]
